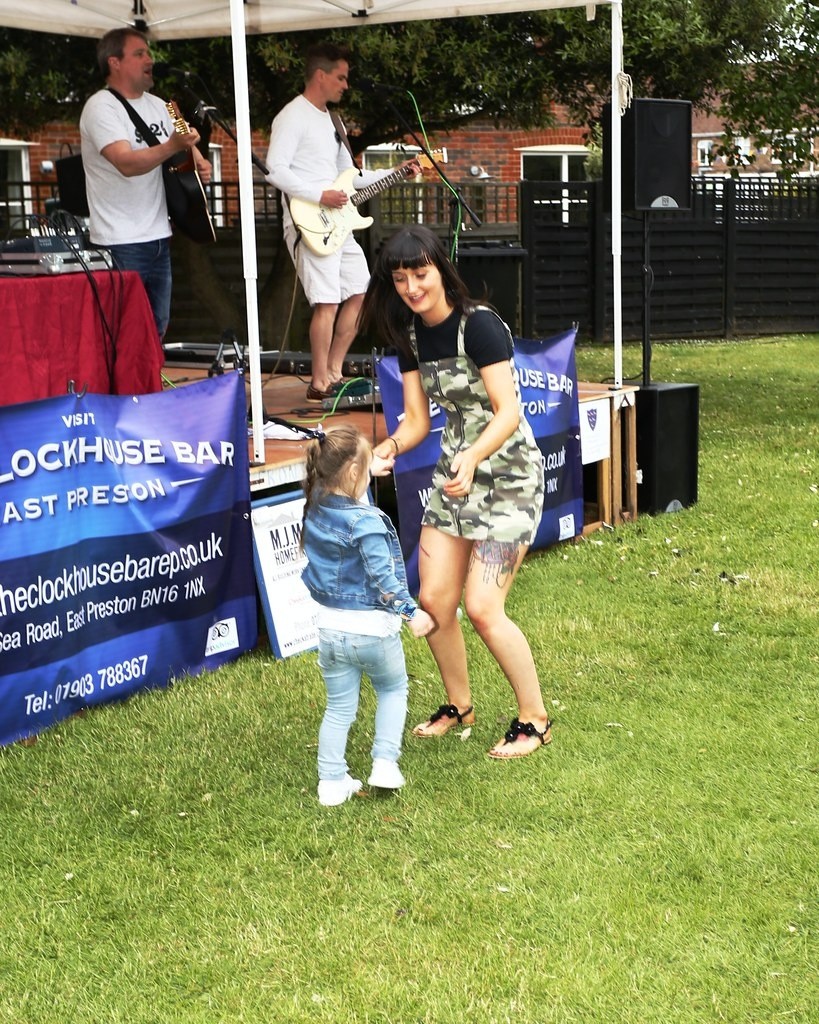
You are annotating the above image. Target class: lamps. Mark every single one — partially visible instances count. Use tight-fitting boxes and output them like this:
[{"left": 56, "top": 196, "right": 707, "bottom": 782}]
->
[{"left": 471, "top": 165, "right": 494, "bottom": 183}]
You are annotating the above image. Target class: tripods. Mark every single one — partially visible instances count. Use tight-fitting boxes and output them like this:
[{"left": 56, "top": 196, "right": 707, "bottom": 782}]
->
[{"left": 182, "top": 83, "right": 327, "bottom": 442}]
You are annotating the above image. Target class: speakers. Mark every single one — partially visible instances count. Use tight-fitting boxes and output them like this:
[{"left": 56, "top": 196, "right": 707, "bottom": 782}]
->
[
  {"left": 622, "top": 98, "right": 693, "bottom": 214},
  {"left": 618, "top": 380, "right": 700, "bottom": 516},
  {"left": 55, "top": 154, "right": 92, "bottom": 219},
  {"left": 454, "top": 248, "right": 532, "bottom": 342}
]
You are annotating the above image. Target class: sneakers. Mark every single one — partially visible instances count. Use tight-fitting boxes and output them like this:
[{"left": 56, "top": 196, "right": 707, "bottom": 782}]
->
[
  {"left": 318, "top": 773, "right": 362, "bottom": 806},
  {"left": 367, "top": 763, "right": 407, "bottom": 789}
]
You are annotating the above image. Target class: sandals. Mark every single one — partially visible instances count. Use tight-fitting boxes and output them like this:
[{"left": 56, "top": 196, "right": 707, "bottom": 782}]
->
[
  {"left": 487, "top": 717, "right": 556, "bottom": 760},
  {"left": 411, "top": 704, "right": 476, "bottom": 738}
]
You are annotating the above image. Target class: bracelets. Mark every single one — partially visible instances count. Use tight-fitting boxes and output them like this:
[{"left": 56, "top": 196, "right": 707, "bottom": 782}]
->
[{"left": 387, "top": 437, "right": 399, "bottom": 456}]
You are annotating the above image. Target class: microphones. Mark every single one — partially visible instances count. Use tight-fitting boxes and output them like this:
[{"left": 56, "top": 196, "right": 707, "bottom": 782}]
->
[{"left": 152, "top": 62, "right": 199, "bottom": 79}]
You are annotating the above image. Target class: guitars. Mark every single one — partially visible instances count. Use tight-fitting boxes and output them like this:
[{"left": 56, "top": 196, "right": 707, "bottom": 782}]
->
[
  {"left": 164, "top": 98, "right": 217, "bottom": 245},
  {"left": 288, "top": 145, "right": 449, "bottom": 258}
]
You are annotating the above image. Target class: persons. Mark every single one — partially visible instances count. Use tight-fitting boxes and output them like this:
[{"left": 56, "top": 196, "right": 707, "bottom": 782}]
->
[
  {"left": 265, "top": 54, "right": 425, "bottom": 399},
  {"left": 371, "top": 226, "right": 552, "bottom": 758},
  {"left": 79, "top": 27, "right": 212, "bottom": 341},
  {"left": 299, "top": 423, "right": 435, "bottom": 806}
]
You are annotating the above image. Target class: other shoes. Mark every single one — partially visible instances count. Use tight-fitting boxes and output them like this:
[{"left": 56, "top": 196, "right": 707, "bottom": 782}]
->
[{"left": 304, "top": 374, "right": 348, "bottom": 404}]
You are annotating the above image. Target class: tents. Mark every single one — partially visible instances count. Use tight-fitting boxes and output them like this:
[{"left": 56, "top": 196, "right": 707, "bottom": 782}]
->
[{"left": 0, "top": 1, "right": 625, "bottom": 458}]
